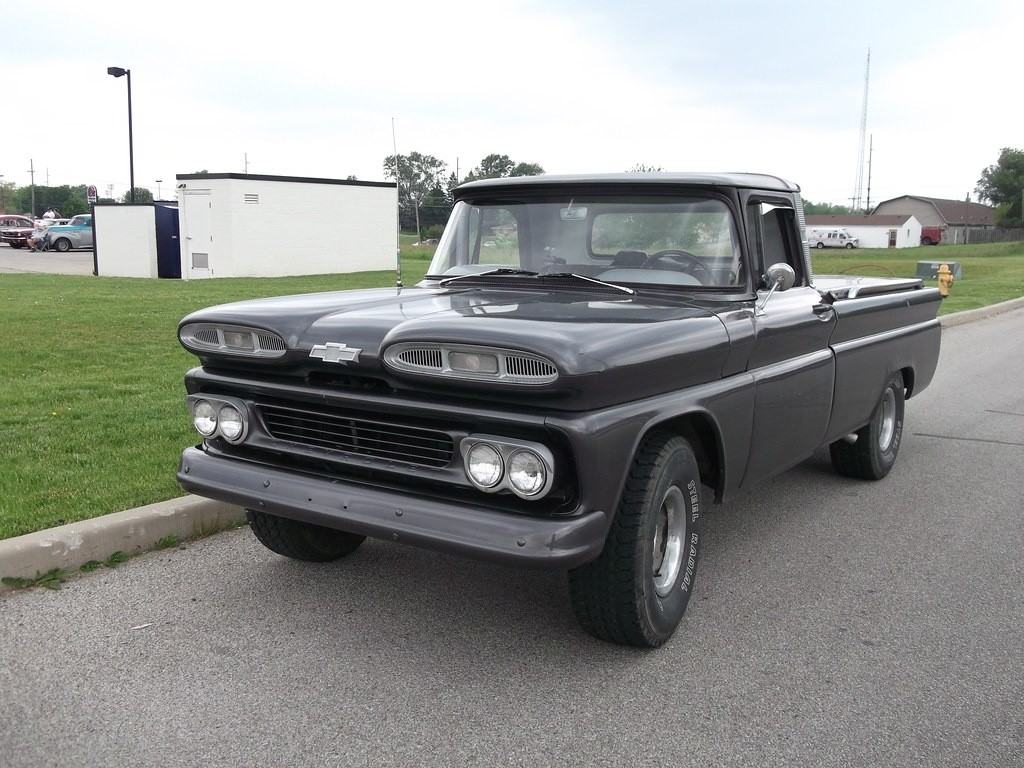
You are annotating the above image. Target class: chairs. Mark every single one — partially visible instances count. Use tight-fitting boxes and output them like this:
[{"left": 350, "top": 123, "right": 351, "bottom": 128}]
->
[{"left": 608, "top": 250, "right": 647, "bottom": 269}]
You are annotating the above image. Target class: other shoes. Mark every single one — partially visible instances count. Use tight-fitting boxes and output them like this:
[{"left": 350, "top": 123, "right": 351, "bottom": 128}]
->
[{"left": 31, "top": 247, "right": 35, "bottom": 252}]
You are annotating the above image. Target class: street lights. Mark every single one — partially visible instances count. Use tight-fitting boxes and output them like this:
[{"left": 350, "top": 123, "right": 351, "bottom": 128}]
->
[
  {"left": 0, "top": 174, "right": 5, "bottom": 210},
  {"left": 107, "top": 66, "right": 135, "bottom": 203},
  {"left": 154, "top": 180, "right": 162, "bottom": 201}
]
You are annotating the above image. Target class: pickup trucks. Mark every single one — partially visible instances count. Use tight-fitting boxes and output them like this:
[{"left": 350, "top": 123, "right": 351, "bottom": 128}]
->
[{"left": 169, "top": 171, "right": 946, "bottom": 650}]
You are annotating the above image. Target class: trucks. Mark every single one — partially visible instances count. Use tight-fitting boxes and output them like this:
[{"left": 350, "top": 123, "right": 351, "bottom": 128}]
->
[{"left": 808, "top": 229, "right": 859, "bottom": 249}]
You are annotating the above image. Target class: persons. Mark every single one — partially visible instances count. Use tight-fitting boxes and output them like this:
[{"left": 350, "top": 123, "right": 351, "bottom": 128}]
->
[
  {"left": 42, "top": 207, "right": 61, "bottom": 218},
  {"left": 26, "top": 223, "right": 48, "bottom": 252}
]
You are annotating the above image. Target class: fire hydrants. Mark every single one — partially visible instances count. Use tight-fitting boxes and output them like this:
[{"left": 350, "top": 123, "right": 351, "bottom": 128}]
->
[{"left": 936, "top": 263, "right": 955, "bottom": 300}]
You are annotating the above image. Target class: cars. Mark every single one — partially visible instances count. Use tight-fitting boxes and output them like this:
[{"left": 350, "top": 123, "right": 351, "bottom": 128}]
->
[
  {"left": 412, "top": 238, "right": 438, "bottom": 248},
  {"left": 45, "top": 214, "right": 93, "bottom": 252},
  {"left": 0, "top": 214, "right": 38, "bottom": 250},
  {"left": 42, "top": 218, "right": 72, "bottom": 233},
  {"left": 484, "top": 241, "right": 496, "bottom": 247}
]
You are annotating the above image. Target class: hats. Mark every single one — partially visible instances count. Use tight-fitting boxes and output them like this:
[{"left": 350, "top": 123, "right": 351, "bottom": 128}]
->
[{"left": 47, "top": 207, "right": 51, "bottom": 210}]
